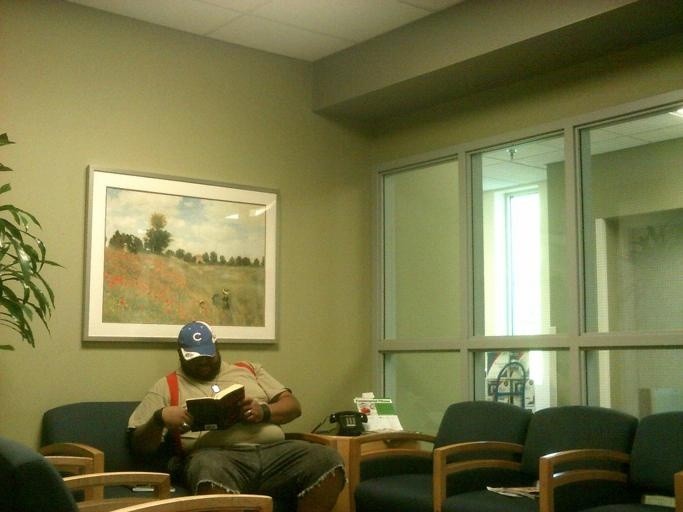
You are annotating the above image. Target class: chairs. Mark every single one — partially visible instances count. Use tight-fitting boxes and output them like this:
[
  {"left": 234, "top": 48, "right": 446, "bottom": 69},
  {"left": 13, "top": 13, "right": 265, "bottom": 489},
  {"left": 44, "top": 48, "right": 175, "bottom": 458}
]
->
[
  {"left": 1, "top": 435, "right": 276, "bottom": 512},
  {"left": 539, "top": 411, "right": 682, "bottom": 511},
  {"left": 350, "top": 401, "right": 533, "bottom": 512},
  {"left": 434, "top": 405, "right": 639, "bottom": 512}
]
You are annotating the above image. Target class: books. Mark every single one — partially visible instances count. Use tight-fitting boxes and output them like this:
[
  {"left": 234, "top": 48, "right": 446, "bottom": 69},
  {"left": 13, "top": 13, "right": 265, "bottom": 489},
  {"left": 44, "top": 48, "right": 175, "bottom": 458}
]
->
[
  {"left": 184, "top": 381, "right": 246, "bottom": 433},
  {"left": 641, "top": 493, "right": 675, "bottom": 508},
  {"left": 486, "top": 485, "right": 541, "bottom": 502},
  {"left": 124, "top": 484, "right": 176, "bottom": 493}
]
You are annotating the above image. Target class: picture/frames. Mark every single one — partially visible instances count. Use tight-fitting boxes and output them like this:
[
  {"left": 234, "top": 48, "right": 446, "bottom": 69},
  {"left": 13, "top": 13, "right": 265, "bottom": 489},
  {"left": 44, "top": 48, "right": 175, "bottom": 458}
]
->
[{"left": 81, "top": 165, "right": 281, "bottom": 346}]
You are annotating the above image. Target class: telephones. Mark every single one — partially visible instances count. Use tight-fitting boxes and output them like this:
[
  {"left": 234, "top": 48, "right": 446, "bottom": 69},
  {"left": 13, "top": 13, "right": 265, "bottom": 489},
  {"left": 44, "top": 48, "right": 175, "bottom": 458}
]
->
[{"left": 329, "top": 409, "right": 369, "bottom": 437}]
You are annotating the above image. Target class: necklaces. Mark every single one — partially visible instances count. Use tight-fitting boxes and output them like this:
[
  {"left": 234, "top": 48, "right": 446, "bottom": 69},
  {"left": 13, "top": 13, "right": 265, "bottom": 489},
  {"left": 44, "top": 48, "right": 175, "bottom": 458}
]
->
[{"left": 206, "top": 371, "right": 219, "bottom": 393}]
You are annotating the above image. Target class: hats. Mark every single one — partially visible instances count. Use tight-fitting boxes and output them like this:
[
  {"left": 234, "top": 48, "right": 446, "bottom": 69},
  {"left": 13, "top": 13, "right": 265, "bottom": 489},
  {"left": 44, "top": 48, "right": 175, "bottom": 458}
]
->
[{"left": 178, "top": 320, "right": 216, "bottom": 362}]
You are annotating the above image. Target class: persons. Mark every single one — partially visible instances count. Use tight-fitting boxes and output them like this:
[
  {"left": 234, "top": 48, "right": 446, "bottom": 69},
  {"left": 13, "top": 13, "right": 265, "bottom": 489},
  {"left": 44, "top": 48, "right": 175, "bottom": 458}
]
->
[{"left": 125, "top": 319, "right": 345, "bottom": 511}]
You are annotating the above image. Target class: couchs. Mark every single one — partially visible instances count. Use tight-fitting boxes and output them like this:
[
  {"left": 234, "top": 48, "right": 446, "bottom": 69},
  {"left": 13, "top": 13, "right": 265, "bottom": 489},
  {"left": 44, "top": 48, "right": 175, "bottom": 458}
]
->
[{"left": 38, "top": 400, "right": 340, "bottom": 512}]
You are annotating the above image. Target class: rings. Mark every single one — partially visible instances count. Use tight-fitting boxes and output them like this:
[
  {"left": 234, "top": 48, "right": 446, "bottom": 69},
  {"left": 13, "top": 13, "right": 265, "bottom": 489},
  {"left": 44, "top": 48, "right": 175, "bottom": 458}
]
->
[{"left": 247, "top": 408, "right": 251, "bottom": 414}]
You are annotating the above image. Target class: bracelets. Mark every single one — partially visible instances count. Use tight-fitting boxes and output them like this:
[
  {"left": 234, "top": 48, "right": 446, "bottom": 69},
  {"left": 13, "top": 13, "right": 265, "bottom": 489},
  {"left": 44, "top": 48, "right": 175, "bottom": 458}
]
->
[
  {"left": 153, "top": 406, "right": 165, "bottom": 428},
  {"left": 259, "top": 402, "right": 271, "bottom": 422}
]
